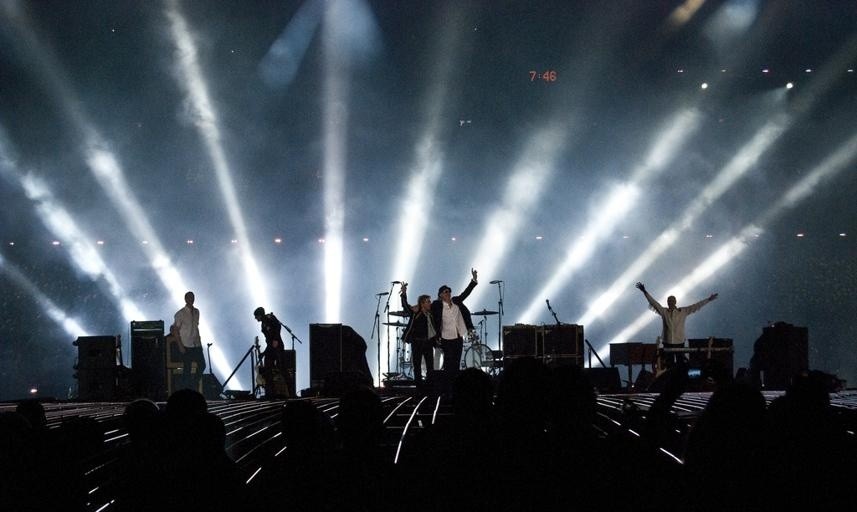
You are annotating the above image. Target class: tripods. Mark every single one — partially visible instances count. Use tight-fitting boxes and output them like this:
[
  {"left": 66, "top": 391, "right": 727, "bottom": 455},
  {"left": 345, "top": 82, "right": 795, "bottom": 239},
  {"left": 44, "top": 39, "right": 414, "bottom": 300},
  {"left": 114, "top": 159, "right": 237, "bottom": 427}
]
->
[
  {"left": 394, "top": 315, "right": 413, "bottom": 380},
  {"left": 478, "top": 302, "right": 504, "bottom": 375}
]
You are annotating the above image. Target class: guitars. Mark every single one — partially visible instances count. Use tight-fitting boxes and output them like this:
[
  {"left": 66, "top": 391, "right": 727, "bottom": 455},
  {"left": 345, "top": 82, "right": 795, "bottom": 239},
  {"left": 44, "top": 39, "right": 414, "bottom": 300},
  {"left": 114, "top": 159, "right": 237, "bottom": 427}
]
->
[{"left": 254, "top": 335, "right": 265, "bottom": 385}]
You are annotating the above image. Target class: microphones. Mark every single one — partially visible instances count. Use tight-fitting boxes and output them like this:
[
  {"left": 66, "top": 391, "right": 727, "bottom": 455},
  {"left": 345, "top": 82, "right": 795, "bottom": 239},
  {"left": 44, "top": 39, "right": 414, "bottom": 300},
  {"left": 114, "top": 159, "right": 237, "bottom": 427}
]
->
[
  {"left": 546, "top": 299, "right": 550, "bottom": 311},
  {"left": 401, "top": 282, "right": 408, "bottom": 289},
  {"left": 490, "top": 280, "right": 502, "bottom": 285},
  {"left": 392, "top": 281, "right": 402, "bottom": 283},
  {"left": 376, "top": 292, "right": 389, "bottom": 295}
]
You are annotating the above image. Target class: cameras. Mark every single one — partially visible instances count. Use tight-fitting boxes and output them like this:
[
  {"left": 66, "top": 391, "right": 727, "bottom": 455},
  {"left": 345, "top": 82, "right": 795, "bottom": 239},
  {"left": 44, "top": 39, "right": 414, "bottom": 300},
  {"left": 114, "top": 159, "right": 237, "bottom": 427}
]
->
[{"left": 687, "top": 367, "right": 702, "bottom": 377}]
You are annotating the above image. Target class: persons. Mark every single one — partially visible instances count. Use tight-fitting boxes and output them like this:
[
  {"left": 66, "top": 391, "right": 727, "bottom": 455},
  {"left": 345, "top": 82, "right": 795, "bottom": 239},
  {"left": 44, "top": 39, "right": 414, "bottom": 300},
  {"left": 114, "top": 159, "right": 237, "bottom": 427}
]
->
[
  {"left": 400, "top": 280, "right": 437, "bottom": 391},
  {"left": 636, "top": 282, "right": 718, "bottom": 392},
  {"left": 164, "top": 324, "right": 184, "bottom": 393},
  {"left": 1, "top": 357, "right": 856, "bottom": 512},
  {"left": 412, "top": 267, "right": 478, "bottom": 388},
  {"left": 254, "top": 307, "right": 300, "bottom": 402},
  {"left": 172, "top": 291, "right": 206, "bottom": 393}
]
